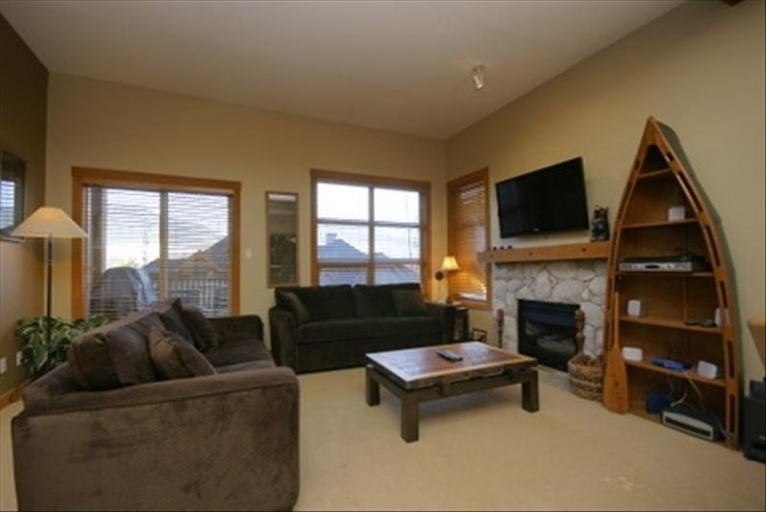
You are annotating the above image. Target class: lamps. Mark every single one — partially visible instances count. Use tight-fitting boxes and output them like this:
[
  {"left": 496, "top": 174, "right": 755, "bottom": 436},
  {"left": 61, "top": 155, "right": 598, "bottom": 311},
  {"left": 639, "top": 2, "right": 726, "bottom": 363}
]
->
[
  {"left": 470, "top": 65, "right": 484, "bottom": 91},
  {"left": 8, "top": 206, "right": 90, "bottom": 348},
  {"left": 441, "top": 256, "right": 460, "bottom": 303}
]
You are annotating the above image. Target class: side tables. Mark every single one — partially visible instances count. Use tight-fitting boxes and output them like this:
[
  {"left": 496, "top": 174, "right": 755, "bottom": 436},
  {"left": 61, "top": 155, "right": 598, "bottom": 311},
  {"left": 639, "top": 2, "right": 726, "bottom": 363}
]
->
[{"left": 425, "top": 301, "right": 468, "bottom": 343}]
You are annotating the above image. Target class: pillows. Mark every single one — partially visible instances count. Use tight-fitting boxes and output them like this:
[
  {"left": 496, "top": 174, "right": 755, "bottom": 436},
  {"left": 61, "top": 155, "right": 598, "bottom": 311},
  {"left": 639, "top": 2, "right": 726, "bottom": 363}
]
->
[
  {"left": 391, "top": 290, "right": 430, "bottom": 317},
  {"left": 68, "top": 298, "right": 218, "bottom": 386},
  {"left": 279, "top": 290, "right": 311, "bottom": 327}
]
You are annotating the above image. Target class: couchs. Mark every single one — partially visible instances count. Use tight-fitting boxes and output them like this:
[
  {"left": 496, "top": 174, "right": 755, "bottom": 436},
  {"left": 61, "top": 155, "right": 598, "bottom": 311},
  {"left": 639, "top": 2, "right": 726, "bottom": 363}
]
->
[
  {"left": 268, "top": 282, "right": 456, "bottom": 374},
  {"left": 9, "top": 297, "right": 300, "bottom": 511}
]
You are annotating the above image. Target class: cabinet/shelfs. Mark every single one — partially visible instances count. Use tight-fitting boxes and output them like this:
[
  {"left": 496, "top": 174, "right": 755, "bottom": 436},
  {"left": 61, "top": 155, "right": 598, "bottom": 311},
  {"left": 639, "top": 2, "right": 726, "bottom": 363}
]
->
[{"left": 603, "top": 116, "right": 742, "bottom": 452}]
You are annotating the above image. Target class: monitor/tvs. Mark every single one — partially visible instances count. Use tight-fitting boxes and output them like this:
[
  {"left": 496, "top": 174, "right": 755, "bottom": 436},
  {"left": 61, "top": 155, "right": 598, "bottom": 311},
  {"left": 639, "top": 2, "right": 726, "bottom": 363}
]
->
[{"left": 495, "top": 157, "right": 590, "bottom": 237}]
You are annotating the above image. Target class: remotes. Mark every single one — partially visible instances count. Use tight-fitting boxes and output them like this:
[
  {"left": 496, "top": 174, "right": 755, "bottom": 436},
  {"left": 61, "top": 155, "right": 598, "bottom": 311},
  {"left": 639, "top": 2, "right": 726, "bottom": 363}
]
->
[{"left": 435, "top": 348, "right": 463, "bottom": 361}]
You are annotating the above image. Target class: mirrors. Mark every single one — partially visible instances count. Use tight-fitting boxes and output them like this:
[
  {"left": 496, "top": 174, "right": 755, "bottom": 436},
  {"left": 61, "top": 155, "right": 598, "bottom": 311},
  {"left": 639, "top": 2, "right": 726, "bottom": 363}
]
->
[{"left": 265, "top": 191, "right": 299, "bottom": 289}]
